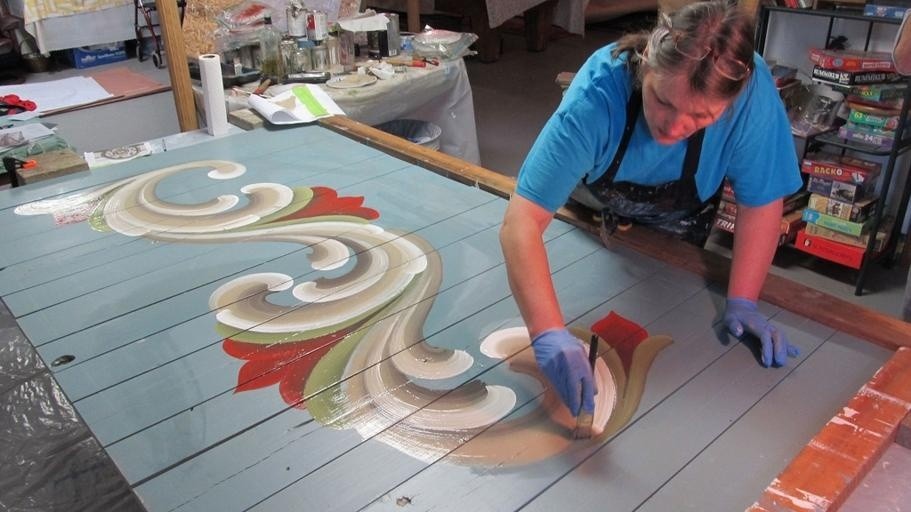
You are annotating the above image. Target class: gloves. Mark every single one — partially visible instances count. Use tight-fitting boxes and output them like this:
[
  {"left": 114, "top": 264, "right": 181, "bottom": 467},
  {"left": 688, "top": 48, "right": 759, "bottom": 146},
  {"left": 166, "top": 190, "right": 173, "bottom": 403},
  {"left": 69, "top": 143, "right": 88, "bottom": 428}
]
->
[
  {"left": 723, "top": 298, "right": 801, "bottom": 369},
  {"left": 531, "top": 328, "right": 599, "bottom": 417}
]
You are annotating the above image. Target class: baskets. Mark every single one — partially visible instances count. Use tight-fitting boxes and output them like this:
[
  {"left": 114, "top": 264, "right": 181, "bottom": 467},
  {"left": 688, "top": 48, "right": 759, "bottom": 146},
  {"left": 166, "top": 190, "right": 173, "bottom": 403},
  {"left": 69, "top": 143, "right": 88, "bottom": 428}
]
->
[{"left": 22, "top": 52, "right": 48, "bottom": 73}]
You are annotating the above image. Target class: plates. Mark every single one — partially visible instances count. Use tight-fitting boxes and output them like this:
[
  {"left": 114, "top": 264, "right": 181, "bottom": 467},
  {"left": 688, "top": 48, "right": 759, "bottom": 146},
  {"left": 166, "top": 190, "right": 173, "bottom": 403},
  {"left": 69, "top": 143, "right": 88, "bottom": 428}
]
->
[{"left": 326, "top": 74, "right": 377, "bottom": 88}]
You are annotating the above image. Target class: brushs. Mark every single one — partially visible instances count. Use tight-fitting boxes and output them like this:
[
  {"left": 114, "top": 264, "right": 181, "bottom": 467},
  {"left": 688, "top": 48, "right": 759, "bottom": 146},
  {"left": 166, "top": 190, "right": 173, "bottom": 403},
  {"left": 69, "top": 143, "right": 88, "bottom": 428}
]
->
[
  {"left": 572, "top": 333, "right": 599, "bottom": 439},
  {"left": 384, "top": 57, "right": 426, "bottom": 67}
]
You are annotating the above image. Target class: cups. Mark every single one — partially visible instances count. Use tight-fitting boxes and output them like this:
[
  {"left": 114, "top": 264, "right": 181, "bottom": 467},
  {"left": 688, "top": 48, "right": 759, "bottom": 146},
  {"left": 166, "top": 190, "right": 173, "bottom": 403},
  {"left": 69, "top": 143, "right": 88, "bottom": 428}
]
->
[{"left": 281, "top": 41, "right": 354, "bottom": 73}]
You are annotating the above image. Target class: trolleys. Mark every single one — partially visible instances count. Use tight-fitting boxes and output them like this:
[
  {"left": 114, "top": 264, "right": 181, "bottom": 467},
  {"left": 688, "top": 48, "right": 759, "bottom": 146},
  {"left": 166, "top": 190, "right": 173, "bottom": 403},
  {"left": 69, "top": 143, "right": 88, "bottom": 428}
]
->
[{"left": 132, "top": 0, "right": 187, "bottom": 68}]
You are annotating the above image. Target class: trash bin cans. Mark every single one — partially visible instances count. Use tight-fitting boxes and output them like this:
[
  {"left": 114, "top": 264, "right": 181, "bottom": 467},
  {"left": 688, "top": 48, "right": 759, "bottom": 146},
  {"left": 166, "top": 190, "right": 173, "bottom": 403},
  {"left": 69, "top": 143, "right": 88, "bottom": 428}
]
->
[{"left": 372, "top": 119, "right": 441, "bottom": 153}]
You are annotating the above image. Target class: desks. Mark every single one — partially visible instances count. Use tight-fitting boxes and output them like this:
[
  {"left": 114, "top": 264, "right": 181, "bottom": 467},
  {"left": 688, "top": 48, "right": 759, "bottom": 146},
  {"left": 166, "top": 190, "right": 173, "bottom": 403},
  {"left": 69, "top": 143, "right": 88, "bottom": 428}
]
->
[
  {"left": 0, "top": 121, "right": 910, "bottom": 510},
  {"left": 246, "top": 64, "right": 481, "bottom": 168}
]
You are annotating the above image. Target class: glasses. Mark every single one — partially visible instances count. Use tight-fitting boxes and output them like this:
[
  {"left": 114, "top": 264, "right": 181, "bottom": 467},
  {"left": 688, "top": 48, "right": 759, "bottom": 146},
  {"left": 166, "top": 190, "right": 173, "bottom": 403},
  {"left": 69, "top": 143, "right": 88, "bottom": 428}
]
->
[{"left": 660, "top": 13, "right": 751, "bottom": 81}]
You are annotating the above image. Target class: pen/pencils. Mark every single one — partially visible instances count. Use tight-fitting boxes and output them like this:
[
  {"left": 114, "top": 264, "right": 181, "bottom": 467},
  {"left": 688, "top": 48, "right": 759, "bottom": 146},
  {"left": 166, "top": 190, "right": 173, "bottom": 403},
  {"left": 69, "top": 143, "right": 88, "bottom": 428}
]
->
[{"left": 162, "top": 139, "right": 166, "bottom": 151}]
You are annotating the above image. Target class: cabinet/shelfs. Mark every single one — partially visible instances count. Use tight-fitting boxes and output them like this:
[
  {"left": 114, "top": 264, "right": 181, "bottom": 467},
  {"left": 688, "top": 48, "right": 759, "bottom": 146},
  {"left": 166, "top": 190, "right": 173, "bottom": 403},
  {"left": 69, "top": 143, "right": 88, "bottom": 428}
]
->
[{"left": 753, "top": 5, "right": 910, "bottom": 292}]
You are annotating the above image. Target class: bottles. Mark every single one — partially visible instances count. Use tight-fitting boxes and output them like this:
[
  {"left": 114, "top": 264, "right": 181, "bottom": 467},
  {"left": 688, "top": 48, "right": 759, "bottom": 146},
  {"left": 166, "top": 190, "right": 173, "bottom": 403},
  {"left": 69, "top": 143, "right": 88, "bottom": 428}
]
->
[{"left": 259, "top": 12, "right": 280, "bottom": 84}]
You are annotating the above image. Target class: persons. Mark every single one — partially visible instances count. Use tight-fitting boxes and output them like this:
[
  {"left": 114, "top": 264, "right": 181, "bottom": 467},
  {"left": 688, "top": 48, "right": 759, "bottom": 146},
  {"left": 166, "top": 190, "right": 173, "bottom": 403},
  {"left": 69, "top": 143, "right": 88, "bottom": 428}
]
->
[
  {"left": 889, "top": 5, "right": 910, "bottom": 76},
  {"left": 497, "top": 1, "right": 807, "bottom": 417}
]
